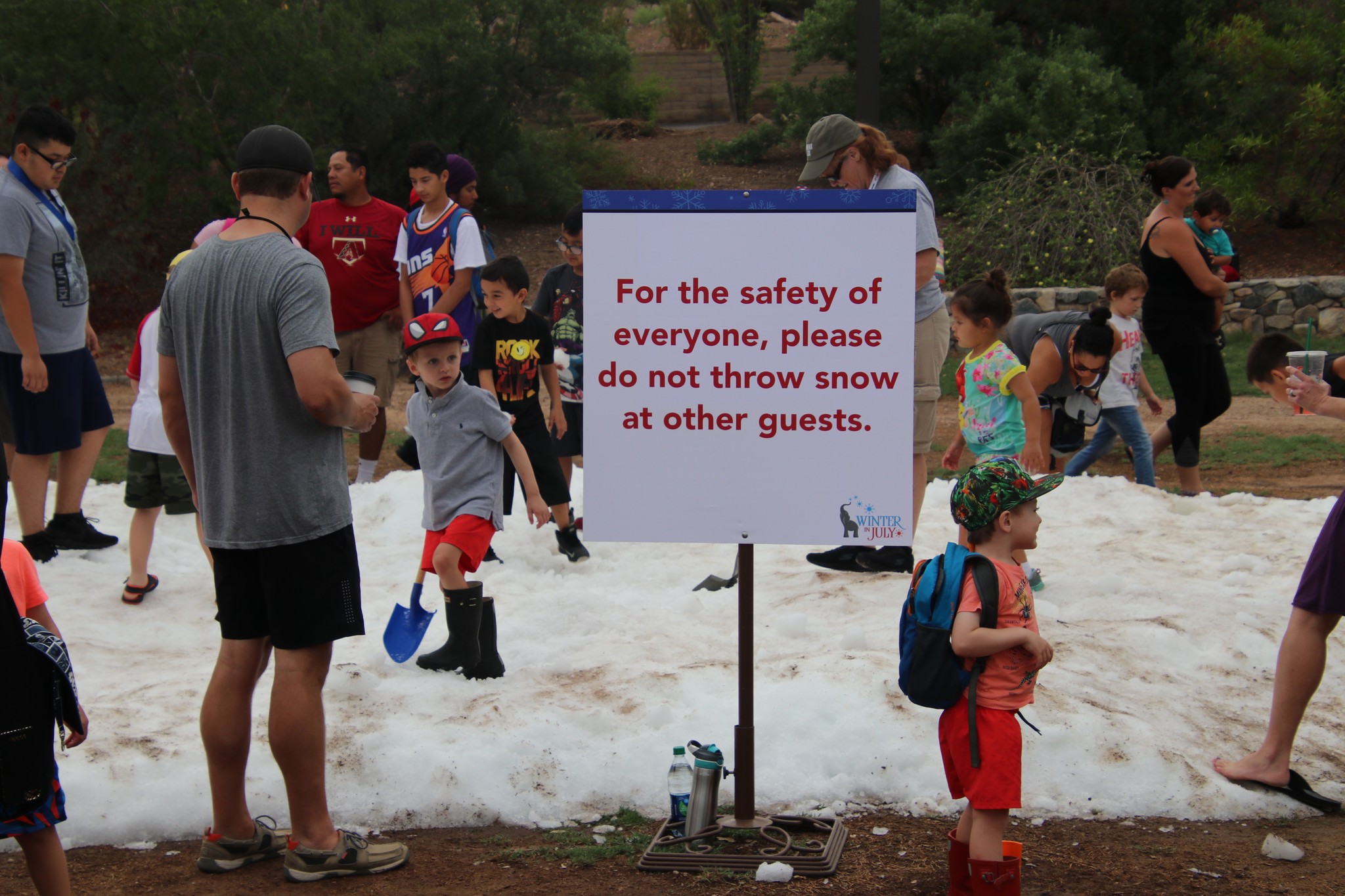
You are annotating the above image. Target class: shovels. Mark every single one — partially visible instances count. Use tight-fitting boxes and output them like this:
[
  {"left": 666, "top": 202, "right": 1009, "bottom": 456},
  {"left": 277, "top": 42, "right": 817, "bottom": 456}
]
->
[{"left": 383, "top": 579, "right": 437, "bottom": 663}]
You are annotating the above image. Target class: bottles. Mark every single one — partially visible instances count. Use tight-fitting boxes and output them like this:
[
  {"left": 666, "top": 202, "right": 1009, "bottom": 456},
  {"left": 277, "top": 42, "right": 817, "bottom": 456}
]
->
[{"left": 668, "top": 746, "right": 693, "bottom": 837}]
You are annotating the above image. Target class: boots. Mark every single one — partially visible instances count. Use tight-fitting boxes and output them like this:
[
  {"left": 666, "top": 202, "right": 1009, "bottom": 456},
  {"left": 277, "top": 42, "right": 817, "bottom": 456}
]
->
[
  {"left": 948, "top": 828, "right": 972, "bottom": 896},
  {"left": 967, "top": 855, "right": 1021, "bottom": 896},
  {"left": 462, "top": 597, "right": 505, "bottom": 679},
  {"left": 416, "top": 580, "right": 483, "bottom": 669}
]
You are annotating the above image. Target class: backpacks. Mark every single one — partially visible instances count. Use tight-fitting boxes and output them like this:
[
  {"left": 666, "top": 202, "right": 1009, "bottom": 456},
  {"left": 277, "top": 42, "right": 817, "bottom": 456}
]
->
[
  {"left": 406, "top": 211, "right": 497, "bottom": 308},
  {"left": 898, "top": 544, "right": 999, "bottom": 711}
]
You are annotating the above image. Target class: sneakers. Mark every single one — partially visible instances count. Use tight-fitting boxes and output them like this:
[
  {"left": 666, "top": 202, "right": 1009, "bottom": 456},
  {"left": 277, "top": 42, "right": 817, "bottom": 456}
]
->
[
  {"left": 46, "top": 510, "right": 118, "bottom": 551},
  {"left": 856, "top": 544, "right": 913, "bottom": 573},
  {"left": 283, "top": 830, "right": 408, "bottom": 882},
  {"left": 807, "top": 545, "right": 877, "bottom": 573},
  {"left": 198, "top": 813, "right": 293, "bottom": 873},
  {"left": 555, "top": 524, "right": 590, "bottom": 562},
  {"left": 21, "top": 533, "right": 58, "bottom": 562}
]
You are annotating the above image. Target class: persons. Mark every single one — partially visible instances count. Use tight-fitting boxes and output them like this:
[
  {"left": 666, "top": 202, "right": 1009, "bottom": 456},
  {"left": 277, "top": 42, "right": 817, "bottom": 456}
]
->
[
  {"left": 1129, "top": 156, "right": 1234, "bottom": 497},
  {"left": 1245, "top": 331, "right": 1345, "bottom": 407},
  {"left": 121, "top": 250, "right": 213, "bottom": 605},
  {"left": 0, "top": 103, "right": 118, "bottom": 564},
  {"left": 937, "top": 456, "right": 1064, "bottom": 896},
  {"left": 941, "top": 264, "right": 1162, "bottom": 490},
  {"left": 157, "top": 123, "right": 404, "bottom": 883},
  {"left": 0, "top": 539, "right": 88, "bottom": 896},
  {"left": 1211, "top": 366, "right": 1345, "bottom": 786},
  {"left": 296, "top": 147, "right": 408, "bottom": 482},
  {"left": 189, "top": 217, "right": 303, "bottom": 251},
  {"left": 393, "top": 144, "right": 589, "bottom": 565},
  {"left": 400, "top": 314, "right": 551, "bottom": 680},
  {"left": 800, "top": 114, "right": 948, "bottom": 572}
]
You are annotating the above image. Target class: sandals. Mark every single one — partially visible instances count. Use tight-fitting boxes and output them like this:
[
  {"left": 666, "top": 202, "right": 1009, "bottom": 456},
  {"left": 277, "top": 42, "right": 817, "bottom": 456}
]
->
[{"left": 121, "top": 574, "right": 159, "bottom": 604}]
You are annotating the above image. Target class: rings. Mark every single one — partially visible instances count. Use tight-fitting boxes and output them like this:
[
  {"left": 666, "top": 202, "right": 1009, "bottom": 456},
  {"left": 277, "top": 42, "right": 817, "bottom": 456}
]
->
[{"left": 1290, "top": 389, "right": 1295, "bottom": 397}]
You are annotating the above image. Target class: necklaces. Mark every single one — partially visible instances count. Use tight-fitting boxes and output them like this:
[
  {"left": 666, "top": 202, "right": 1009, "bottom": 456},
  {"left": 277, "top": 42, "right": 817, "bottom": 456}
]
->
[{"left": 234, "top": 208, "right": 293, "bottom": 245}]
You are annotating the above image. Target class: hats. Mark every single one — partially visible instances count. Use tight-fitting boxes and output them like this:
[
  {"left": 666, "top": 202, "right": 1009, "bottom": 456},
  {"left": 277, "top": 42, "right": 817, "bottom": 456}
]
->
[
  {"left": 445, "top": 154, "right": 476, "bottom": 193},
  {"left": 950, "top": 458, "right": 1064, "bottom": 530},
  {"left": 799, "top": 114, "right": 863, "bottom": 182},
  {"left": 166, "top": 249, "right": 196, "bottom": 281},
  {"left": 236, "top": 126, "right": 321, "bottom": 203},
  {"left": 404, "top": 313, "right": 464, "bottom": 354}
]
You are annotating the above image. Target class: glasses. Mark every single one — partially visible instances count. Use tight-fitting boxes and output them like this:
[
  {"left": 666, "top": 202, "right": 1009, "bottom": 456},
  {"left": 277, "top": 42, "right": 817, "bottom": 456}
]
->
[
  {"left": 556, "top": 236, "right": 582, "bottom": 255},
  {"left": 820, "top": 155, "right": 848, "bottom": 181},
  {"left": 25, "top": 145, "right": 76, "bottom": 170},
  {"left": 1072, "top": 350, "right": 1108, "bottom": 374}
]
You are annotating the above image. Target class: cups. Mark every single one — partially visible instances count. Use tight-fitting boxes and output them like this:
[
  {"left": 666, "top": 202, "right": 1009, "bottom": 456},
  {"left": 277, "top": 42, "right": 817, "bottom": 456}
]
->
[
  {"left": 1286, "top": 351, "right": 1328, "bottom": 415},
  {"left": 343, "top": 370, "right": 378, "bottom": 432}
]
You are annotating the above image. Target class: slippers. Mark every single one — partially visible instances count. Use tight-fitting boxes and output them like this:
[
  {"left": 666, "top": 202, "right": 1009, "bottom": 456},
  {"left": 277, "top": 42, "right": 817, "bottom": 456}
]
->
[
  {"left": 1125, "top": 447, "right": 1136, "bottom": 465},
  {"left": 1254, "top": 768, "right": 1341, "bottom": 813}
]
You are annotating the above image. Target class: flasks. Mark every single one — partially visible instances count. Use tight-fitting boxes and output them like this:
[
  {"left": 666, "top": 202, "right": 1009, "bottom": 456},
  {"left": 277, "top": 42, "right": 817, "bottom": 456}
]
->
[{"left": 686, "top": 740, "right": 724, "bottom": 843}]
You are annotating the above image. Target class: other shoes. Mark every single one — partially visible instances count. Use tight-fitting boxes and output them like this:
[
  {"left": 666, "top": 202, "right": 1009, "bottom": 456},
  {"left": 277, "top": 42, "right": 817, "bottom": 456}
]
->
[{"left": 1027, "top": 568, "right": 1044, "bottom": 591}]
